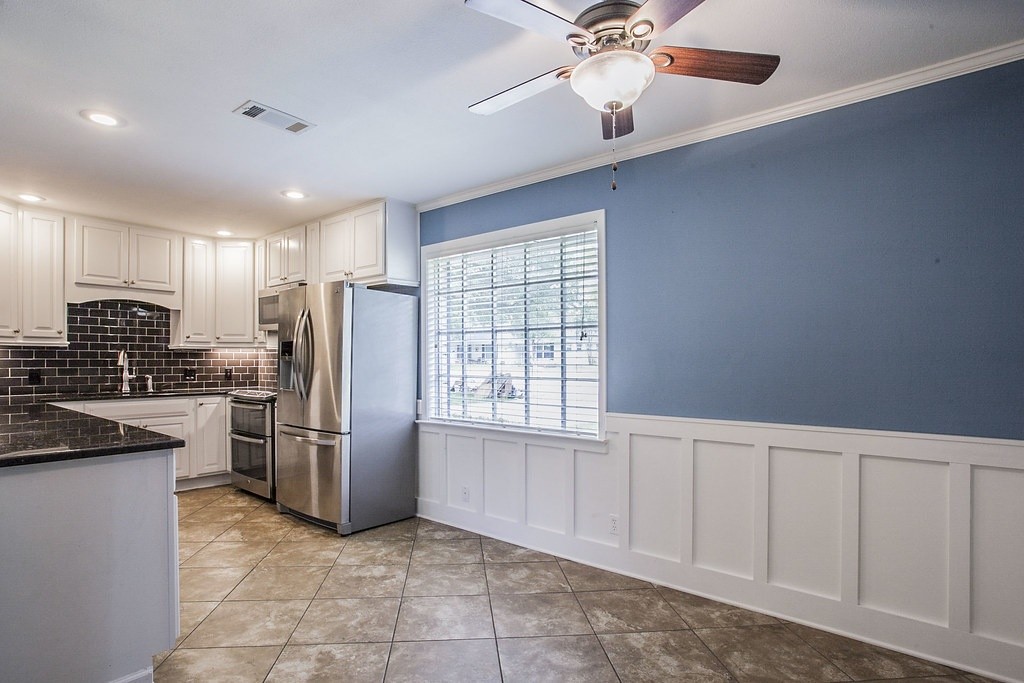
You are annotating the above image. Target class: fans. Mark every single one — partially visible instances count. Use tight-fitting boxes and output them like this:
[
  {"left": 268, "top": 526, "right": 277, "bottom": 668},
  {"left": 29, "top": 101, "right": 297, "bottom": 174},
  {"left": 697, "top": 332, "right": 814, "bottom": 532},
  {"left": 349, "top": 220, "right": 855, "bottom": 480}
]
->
[{"left": 466, "top": 0, "right": 781, "bottom": 140}]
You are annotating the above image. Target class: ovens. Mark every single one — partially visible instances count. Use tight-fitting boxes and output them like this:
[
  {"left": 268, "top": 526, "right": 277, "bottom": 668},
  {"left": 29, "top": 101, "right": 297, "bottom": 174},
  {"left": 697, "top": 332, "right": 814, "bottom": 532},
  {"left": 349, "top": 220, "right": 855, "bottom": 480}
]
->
[{"left": 228, "top": 397, "right": 275, "bottom": 503}]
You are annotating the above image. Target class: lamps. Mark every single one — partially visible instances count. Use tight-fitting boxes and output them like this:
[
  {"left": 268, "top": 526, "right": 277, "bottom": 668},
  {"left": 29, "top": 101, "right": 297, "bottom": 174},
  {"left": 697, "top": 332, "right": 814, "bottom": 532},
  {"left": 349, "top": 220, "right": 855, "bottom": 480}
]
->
[
  {"left": 570, "top": 39, "right": 656, "bottom": 192},
  {"left": 78, "top": 108, "right": 129, "bottom": 130}
]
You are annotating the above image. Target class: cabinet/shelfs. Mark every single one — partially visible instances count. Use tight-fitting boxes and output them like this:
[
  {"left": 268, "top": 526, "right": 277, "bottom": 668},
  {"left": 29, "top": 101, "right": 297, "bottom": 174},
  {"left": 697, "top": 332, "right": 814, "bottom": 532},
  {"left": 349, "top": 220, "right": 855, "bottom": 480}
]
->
[
  {"left": 66, "top": 215, "right": 181, "bottom": 311},
  {"left": 0, "top": 201, "right": 71, "bottom": 346},
  {"left": 0, "top": 449, "right": 182, "bottom": 683},
  {"left": 168, "top": 232, "right": 259, "bottom": 350},
  {"left": 320, "top": 200, "right": 420, "bottom": 288},
  {"left": 84, "top": 395, "right": 193, "bottom": 482},
  {"left": 265, "top": 225, "right": 307, "bottom": 289},
  {"left": 192, "top": 395, "right": 226, "bottom": 478}
]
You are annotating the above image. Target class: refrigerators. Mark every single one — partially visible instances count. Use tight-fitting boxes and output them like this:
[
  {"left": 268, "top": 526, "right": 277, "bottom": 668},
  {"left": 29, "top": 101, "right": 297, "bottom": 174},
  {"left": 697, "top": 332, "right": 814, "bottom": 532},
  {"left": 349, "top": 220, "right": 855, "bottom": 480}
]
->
[{"left": 275, "top": 281, "right": 417, "bottom": 535}]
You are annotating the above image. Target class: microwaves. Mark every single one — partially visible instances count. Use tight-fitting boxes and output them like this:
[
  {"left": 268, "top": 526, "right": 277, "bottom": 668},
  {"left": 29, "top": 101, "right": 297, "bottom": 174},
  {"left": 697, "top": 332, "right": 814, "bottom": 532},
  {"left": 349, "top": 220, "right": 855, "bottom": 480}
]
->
[{"left": 258, "top": 283, "right": 306, "bottom": 330}]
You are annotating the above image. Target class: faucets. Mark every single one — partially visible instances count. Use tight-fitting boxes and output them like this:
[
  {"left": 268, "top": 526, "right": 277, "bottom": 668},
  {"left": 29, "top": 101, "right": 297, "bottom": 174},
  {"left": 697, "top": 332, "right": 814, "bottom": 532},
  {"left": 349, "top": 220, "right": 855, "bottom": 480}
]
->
[{"left": 116, "top": 347, "right": 129, "bottom": 393}]
